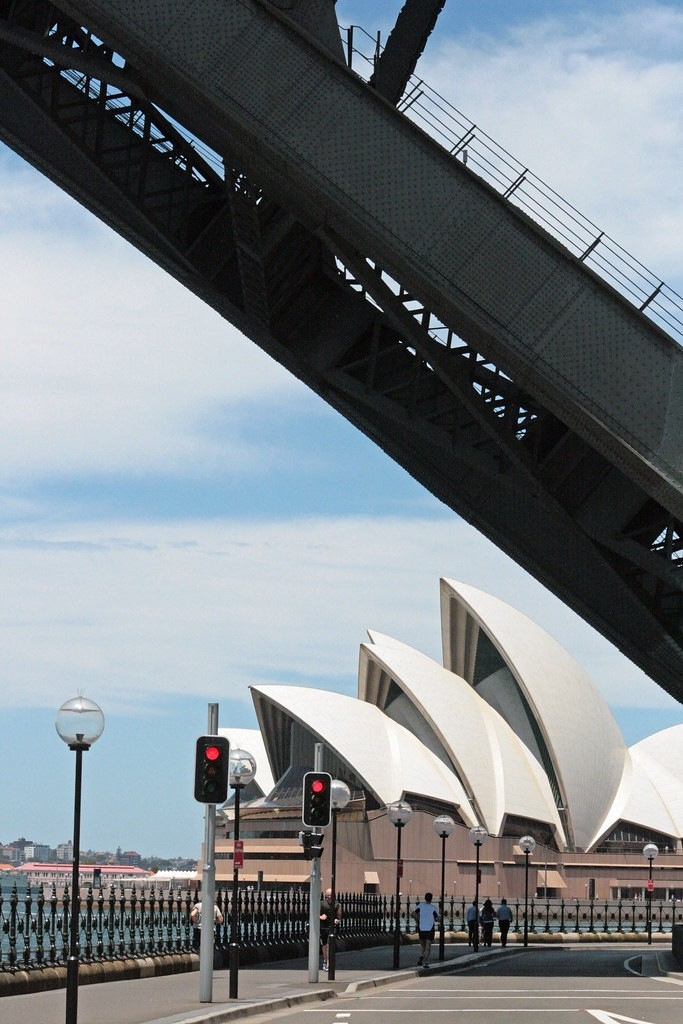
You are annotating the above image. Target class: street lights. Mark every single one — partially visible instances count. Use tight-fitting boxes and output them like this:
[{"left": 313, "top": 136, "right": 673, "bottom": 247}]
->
[
  {"left": 228, "top": 749, "right": 257, "bottom": 1002},
  {"left": 327, "top": 778, "right": 352, "bottom": 981},
  {"left": 518, "top": 836, "right": 536, "bottom": 947},
  {"left": 468, "top": 826, "right": 489, "bottom": 952},
  {"left": 387, "top": 800, "right": 413, "bottom": 971},
  {"left": 433, "top": 815, "right": 456, "bottom": 961},
  {"left": 54, "top": 698, "right": 106, "bottom": 1024},
  {"left": 643, "top": 844, "right": 659, "bottom": 946}
]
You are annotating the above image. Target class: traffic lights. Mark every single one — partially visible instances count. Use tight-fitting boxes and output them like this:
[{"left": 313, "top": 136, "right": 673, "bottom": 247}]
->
[
  {"left": 194, "top": 735, "right": 230, "bottom": 805},
  {"left": 304, "top": 832, "right": 325, "bottom": 861},
  {"left": 301, "top": 773, "right": 332, "bottom": 828}
]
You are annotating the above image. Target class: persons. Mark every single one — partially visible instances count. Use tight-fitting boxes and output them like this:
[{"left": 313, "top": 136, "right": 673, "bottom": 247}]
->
[
  {"left": 412, "top": 892, "right": 439, "bottom": 967},
  {"left": 467, "top": 901, "right": 479, "bottom": 947},
  {"left": 496, "top": 899, "right": 512, "bottom": 948},
  {"left": 190, "top": 902, "right": 224, "bottom": 954},
  {"left": 320, "top": 888, "right": 340, "bottom": 972},
  {"left": 480, "top": 900, "right": 495, "bottom": 947}
]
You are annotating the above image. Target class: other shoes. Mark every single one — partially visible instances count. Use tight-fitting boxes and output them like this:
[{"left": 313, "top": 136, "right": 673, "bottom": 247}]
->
[
  {"left": 488, "top": 945, "right": 491, "bottom": 947},
  {"left": 423, "top": 966, "right": 429, "bottom": 969},
  {"left": 469, "top": 945, "right": 471, "bottom": 946},
  {"left": 473, "top": 945, "right": 475, "bottom": 946},
  {"left": 502, "top": 945, "right": 506, "bottom": 947},
  {"left": 323, "top": 963, "right": 330, "bottom": 972},
  {"left": 417, "top": 961, "right": 423, "bottom": 966},
  {"left": 484, "top": 945, "right": 486, "bottom": 946}
]
